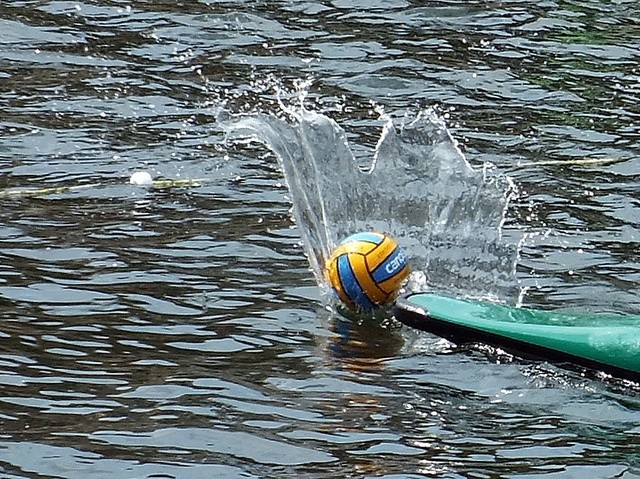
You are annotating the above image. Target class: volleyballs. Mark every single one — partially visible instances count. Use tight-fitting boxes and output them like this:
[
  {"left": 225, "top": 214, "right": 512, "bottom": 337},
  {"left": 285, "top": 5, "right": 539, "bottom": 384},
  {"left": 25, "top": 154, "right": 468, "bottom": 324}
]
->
[{"left": 325, "top": 232, "right": 411, "bottom": 312}]
